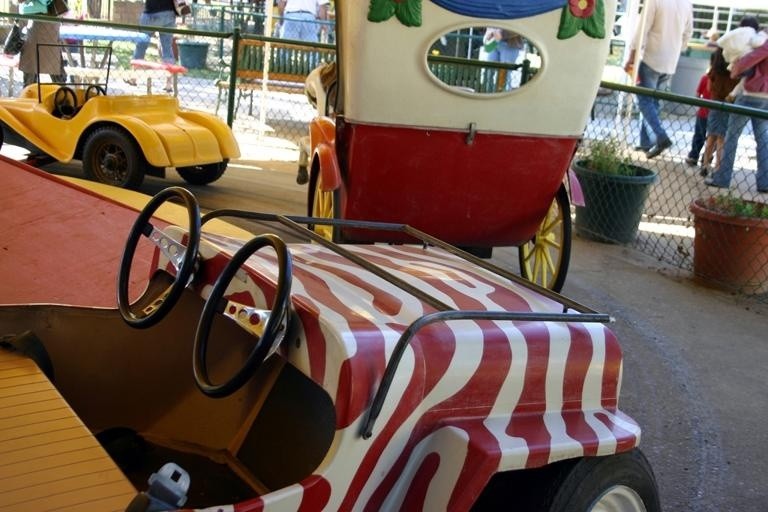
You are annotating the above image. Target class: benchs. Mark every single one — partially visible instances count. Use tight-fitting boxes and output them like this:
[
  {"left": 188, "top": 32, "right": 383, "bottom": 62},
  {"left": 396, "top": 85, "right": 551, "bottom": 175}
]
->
[
  {"left": 213, "top": 39, "right": 337, "bottom": 130},
  {"left": 0, "top": 51, "right": 119, "bottom": 68},
  {"left": 130, "top": 58, "right": 189, "bottom": 98},
  {"left": 0, "top": 339, "right": 140, "bottom": 512},
  {"left": 63, "top": 66, "right": 171, "bottom": 96}
]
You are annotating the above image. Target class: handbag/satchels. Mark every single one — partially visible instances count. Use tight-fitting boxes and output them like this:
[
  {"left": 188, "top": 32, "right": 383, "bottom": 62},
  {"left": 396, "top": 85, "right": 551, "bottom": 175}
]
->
[
  {"left": 4, "top": 23, "right": 25, "bottom": 58},
  {"left": 48, "top": 0, "right": 69, "bottom": 16},
  {"left": 174, "top": 1, "right": 191, "bottom": 17}
]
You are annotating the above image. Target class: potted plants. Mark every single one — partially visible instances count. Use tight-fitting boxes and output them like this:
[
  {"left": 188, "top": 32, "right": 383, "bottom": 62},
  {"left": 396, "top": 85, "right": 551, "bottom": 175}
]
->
[
  {"left": 572, "top": 140, "right": 657, "bottom": 246},
  {"left": 687, "top": 194, "right": 768, "bottom": 296}
]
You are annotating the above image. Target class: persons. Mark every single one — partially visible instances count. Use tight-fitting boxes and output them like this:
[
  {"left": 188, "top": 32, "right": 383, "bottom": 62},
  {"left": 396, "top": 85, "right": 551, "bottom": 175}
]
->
[
  {"left": 624, "top": 0, "right": 693, "bottom": 159},
  {"left": 685, "top": 15, "right": 768, "bottom": 192},
  {"left": 124, "top": 0, "right": 177, "bottom": 92},
  {"left": 484, "top": 27, "right": 523, "bottom": 92},
  {"left": 19, "top": 0, "right": 67, "bottom": 88},
  {"left": 276, "top": 0, "right": 320, "bottom": 69}
]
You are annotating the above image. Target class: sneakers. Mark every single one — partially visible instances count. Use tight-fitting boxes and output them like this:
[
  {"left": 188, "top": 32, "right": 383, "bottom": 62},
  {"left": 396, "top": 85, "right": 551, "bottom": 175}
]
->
[{"left": 685, "top": 153, "right": 720, "bottom": 178}]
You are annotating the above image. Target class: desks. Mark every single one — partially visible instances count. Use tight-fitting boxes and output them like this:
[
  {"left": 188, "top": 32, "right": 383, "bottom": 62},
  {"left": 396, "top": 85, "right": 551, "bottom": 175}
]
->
[{"left": 21, "top": 24, "right": 149, "bottom": 91}]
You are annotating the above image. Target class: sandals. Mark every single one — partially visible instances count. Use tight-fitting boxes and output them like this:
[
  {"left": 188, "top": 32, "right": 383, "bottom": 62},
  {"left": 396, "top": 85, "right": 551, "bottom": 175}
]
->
[
  {"left": 162, "top": 88, "right": 173, "bottom": 93},
  {"left": 123, "top": 78, "right": 138, "bottom": 86}
]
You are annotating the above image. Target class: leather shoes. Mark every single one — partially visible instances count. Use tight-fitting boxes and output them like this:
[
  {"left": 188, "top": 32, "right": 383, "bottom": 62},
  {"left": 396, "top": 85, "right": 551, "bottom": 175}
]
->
[
  {"left": 757, "top": 187, "right": 768, "bottom": 193},
  {"left": 645, "top": 140, "right": 672, "bottom": 159},
  {"left": 635, "top": 145, "right": 651, "bottom": 152},
  {"left": 705, "top": 180, "right": 728, "bottom": 188}
]
